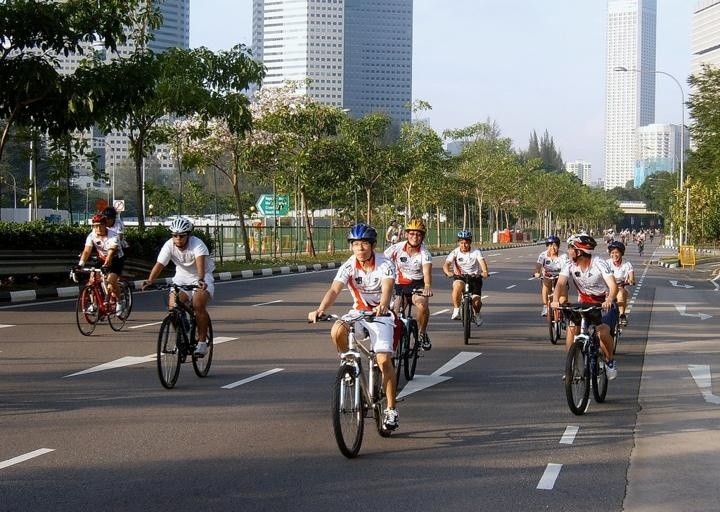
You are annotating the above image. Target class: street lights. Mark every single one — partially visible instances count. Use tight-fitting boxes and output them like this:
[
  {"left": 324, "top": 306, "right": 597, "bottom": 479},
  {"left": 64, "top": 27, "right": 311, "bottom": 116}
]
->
[{"left": 612, "top": 64, "right": 685, "bottom": 257}]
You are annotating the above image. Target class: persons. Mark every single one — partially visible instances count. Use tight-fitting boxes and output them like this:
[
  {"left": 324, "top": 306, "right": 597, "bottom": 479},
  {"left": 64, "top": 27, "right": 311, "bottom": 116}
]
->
[
  {"left": 307, "top": 224, "right": 399, "bottom": 430},
  {"left": 385, "top": 218, "right": 401, "bottom": 247},
  {"left": 548, "top": 234, "right": 619, "bottom": 381},
  {"left": 68, "top": 213, "right": 125, "bottom": 318},
  {"left": 565, "top": 227, "right": 662, "bottom": 257},
  {"left": 101, "top": 207, "right": 130, "bottom": 282},
  {"left": 604, "top": 240, "right": 636, "bottom": 328},
  {"left": 141, "top": 216, "right": 215, "bottom": 357},
  {"left": 533, "top": 235, "right": 573, "bottom": 317},
  {"left": 441, "top": 230, "right": 488, "bottom": 328},
  {"left": 382, "top": 219, "right": 433, "bottom": 351}
]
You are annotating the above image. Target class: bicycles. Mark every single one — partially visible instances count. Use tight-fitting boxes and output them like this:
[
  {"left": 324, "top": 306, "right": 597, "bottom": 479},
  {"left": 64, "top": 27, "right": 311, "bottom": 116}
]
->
[
  {"left": 64, "top": 263, "right": 135, "bottom": 325},
  {"left": 447, "top": 272, "right": 490, "bottom": 344},
  {"left": 70, "top": 267, "right": 129, "bottom": 336},
  {"left": 532, "top": 272, "right": 570, "bottom": 344},
  {"left": 554, "top": 300, "right": 615, "bottom": 416},
  {"left": 392, "top": 288, "right": 432, "bottom": 388},
  {"left": 144, "top": 278, "right": 215, "bottom": 389},
  {"left": 309, "top": 310, "right": 405, "bottom": 459},
  {"left": 613, "top": 281, "right": 635, "bottom": 354}
]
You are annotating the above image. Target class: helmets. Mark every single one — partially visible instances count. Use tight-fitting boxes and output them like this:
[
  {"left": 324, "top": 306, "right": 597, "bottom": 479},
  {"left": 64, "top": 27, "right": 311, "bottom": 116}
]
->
[
  {"left": 545, "top": 235, "right": 560, "bottom": 245},
  {"left": 568, "top": 233, "right": 596, "bottom": 254},
  {"left": 169, "top": 216, "right": 192, "bottom": 234},
  {"left": 456, "top": 230, "right": 472, "bottom": 240},
  {"left": 91, "top": 208, "right": 116, "bottom": 226},
  {"left": 346, "top": 224, "right": 378, "bottom": 241},
  {"left": 608, "top": 241, "right": 625, "bottom": 252},
  {"left": 405, "top": 219, "right": 426, "bottom": 233}
]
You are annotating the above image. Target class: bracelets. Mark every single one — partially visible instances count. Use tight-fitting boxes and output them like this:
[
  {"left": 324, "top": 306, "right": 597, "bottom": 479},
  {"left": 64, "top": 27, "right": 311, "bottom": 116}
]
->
[{"left": 197, "top": 278, "right": 207, "bottom": 282}]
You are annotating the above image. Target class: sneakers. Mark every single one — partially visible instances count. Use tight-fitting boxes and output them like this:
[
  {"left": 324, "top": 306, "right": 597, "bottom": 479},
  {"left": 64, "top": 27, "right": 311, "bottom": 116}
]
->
[
  {"left": 621, "top": 314, "right": 627, "bottom": 324},
  {"left": 383, "top": 406, "right": 400, "bottom": 434},
  {"left": 475, "top": 312, "right": 483, "bottom": 327},
  {"left": 451, "top": 308, "right": 461, "bottom": 320},
  {"left": 194, "top": 342, "right": 208, "bottom": 355},
  {"left": 419, "top": 332, "right": 431, "bottom": 353},
  {"left": 603, "top": 359, "right": 617, "bottom": 380},
  {"left": 88, "top": 304, "right": 97, "bottom": 313},
  {"left": 540, "top": 305, "right": 549, "bottom": 317},
  {"left": 115, "top": 300, "right": 126, "bottom": 316}
]
